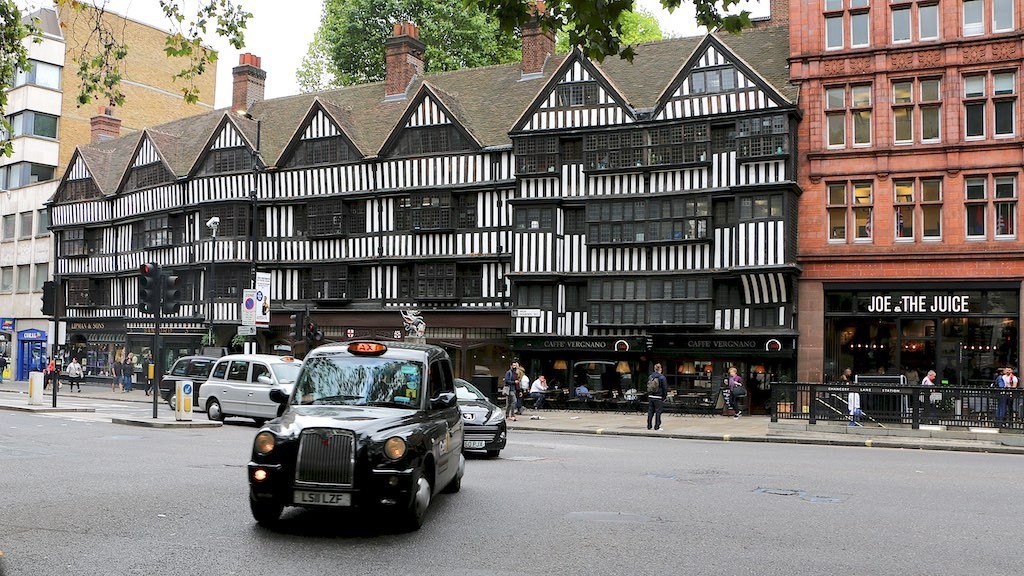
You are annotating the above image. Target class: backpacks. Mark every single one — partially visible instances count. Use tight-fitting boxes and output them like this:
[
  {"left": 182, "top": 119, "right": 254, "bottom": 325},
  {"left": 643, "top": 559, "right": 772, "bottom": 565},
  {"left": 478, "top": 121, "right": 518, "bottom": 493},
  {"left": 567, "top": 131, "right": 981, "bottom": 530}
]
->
[
  {"left": 989, "top": 378, "right": 1001, "bottom": 399},
  {"left": 1009, "top": 374, "right": 1021, "bottom": 386},
  {"left": 646, "top": 375, "right": 660, "bottom": 394}
]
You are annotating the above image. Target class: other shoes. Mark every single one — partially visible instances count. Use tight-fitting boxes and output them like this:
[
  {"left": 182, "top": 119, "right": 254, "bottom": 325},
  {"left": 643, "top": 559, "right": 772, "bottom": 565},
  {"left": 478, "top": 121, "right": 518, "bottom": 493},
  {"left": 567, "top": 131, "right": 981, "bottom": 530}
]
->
[
  {"left": 506, "top": 416, "right": 514, "bottom": 421},
  {"left": 733, "top": 411, "right": 742, "bottom": 419},
  {"left": 647, "top": 425, "right": 652, "bottom": 429},
  {"left": 515, "top": 406, "right": 527, "bottom": 414},
  {"left": 511, "top": 416, "right": 516, "bottom": 421},
  {"left": 653, "top": 427, "right": 664, "bottom": 430}
]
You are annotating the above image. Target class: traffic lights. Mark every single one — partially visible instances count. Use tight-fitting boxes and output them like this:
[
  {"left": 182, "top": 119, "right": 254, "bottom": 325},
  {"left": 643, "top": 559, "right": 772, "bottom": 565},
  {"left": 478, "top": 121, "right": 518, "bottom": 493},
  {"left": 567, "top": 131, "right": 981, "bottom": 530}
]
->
[
  {"left": 163, "top": 274, "right": 181, "bottom": 314},
  {"left": 138, "top": 262, "right": 158, "bottom": 314}
]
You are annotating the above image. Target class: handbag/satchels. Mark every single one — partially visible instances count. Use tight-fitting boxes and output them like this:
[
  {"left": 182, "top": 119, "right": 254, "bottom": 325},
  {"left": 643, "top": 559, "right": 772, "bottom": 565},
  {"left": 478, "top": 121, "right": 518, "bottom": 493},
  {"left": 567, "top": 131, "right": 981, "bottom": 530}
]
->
[
  {"left": 501, "top": 384, "right": 510, "bottom": 395},
  {"left": 733, "top": 383, "right": 747, "bottom": 398},
  {"left": 518, "top": 389, "right": 524, "bottom": 398}
]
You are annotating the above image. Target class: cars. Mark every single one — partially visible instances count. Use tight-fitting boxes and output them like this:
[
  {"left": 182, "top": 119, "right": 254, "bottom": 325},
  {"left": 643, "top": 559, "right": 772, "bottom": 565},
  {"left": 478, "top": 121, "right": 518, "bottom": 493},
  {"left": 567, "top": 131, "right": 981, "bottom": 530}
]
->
[
  {"left": 455, "top": 378, "right": 507, "bottom": 458},
  {"left": 159, "top": 356, "right": 219, "bottom": 411},
  {"left": 198, "top": 354, "right": 303, "bottom": 425}
]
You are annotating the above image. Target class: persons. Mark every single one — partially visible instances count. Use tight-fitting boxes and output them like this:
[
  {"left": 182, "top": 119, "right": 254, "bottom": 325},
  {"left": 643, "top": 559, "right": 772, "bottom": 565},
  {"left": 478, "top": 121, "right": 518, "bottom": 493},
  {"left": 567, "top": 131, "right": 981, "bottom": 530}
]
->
[
  {"left": 574, "top": 382, "right": 594, "bottom": 398},
  {"left": 65, "top": 357, "right": 85, "bottom": 393},
  {"left": 840, "top": 366, "right": 936, "bottom": 386},
  {"left": 261, "top": 295, "right": 270, "bottom": 316},
  {"left": 529, "top": 375, "right": 549, "bottom": 408},
  {"left": 111, "top": 353, "right": 154, "bottom": 396},
  {"left": 646, "top": 363, "right": 669, "bottom": 431},
  {"left": 0, "top": 352, "right": 9, "bottom": 386},
  {"left": 992, "top": 366, "right": 1019, "bottom": 427},
  {"left": 42, "top": 357, "right": 63, "bottom": 390},
  {"left": 724, "top": 367, "right": 743, "bottom": 420},
  {"left": 504, "top": 361, "right": 529, "bottom": 421}
]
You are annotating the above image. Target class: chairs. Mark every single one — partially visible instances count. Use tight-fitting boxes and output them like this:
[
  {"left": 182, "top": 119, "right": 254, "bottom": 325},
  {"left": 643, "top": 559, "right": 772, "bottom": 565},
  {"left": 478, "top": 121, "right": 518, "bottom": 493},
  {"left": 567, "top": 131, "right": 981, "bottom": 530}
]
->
[{"left": 497, "top": 395, "right": 717, "bottom": 419}]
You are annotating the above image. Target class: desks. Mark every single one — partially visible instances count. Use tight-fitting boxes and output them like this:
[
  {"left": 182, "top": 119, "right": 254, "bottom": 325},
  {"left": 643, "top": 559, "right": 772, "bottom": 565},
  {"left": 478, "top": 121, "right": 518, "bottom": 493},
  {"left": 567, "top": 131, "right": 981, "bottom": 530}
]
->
[{"left": 537, "top": 390, "right": 700, "bottom": 412}]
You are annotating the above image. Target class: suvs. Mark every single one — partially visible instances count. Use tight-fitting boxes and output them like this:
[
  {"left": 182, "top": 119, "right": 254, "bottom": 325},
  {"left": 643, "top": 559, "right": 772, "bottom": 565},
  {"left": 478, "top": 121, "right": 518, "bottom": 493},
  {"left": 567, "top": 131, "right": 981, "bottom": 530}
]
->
[{"left": 248, "top": 339, "right": 464, "bottom": 534}]
